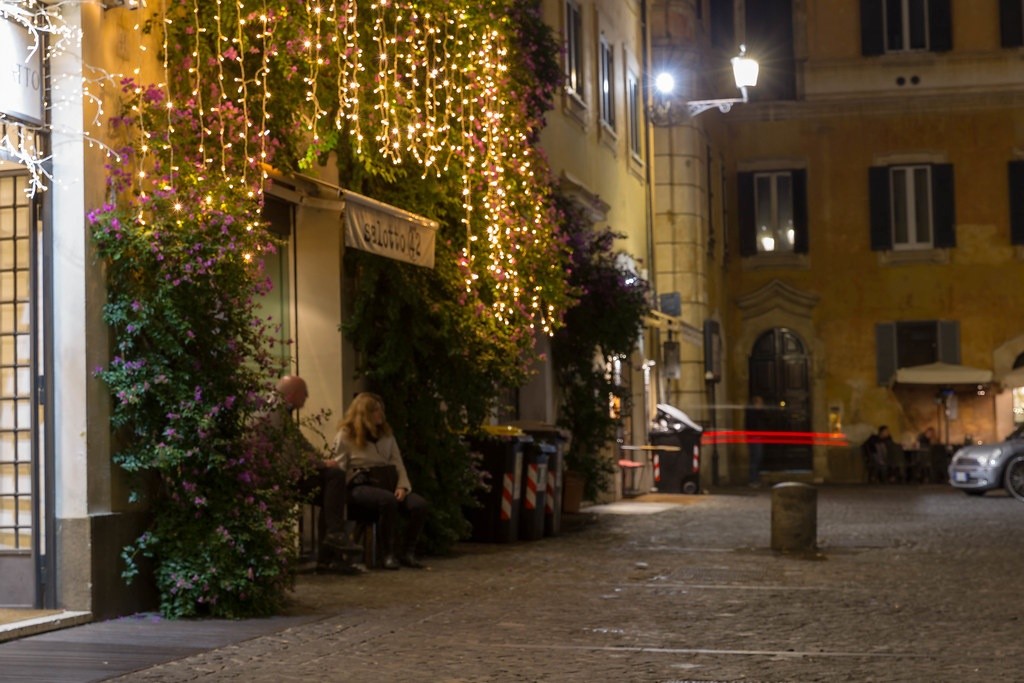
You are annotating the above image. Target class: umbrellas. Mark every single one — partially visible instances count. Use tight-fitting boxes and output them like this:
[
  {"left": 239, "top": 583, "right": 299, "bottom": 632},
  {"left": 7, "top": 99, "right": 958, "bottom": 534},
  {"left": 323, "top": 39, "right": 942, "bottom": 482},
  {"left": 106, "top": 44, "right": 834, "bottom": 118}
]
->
[
  {"left": 896, "top": 361, "right": 1002, "bottom": 446},
  {"left": 1002, "top": 366, "right": 1024, "bottom": 388}
]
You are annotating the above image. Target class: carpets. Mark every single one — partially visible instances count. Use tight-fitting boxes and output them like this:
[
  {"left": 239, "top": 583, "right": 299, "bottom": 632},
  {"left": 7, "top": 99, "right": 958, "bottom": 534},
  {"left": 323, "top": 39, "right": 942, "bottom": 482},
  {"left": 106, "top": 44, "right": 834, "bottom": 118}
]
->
[{"left": 0, "top": 606, "right": 339, "bottom": 683}]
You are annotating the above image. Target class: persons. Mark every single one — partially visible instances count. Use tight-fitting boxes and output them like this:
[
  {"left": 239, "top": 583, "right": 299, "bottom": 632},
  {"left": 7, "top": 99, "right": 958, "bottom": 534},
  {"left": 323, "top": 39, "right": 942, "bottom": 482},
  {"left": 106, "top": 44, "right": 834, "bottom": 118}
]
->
[
  {"left": 916, "top": 428, "right": 941, "bottom": 448},
  {"left": 328, "top": 391, "right": 425, "bottom": 571},
  {"left": 869, "top": 426, "right": 909, "bottom": 485},
  {"left": 270, "top": 376, "right": 360, "bottom": 575}
]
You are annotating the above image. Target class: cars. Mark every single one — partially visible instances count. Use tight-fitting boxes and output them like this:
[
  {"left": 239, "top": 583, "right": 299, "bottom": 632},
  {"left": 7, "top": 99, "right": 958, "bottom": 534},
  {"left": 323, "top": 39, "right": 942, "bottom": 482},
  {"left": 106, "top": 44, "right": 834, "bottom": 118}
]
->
[{"left": 948, "top": 423, "right": 1024, "bottom": 499}]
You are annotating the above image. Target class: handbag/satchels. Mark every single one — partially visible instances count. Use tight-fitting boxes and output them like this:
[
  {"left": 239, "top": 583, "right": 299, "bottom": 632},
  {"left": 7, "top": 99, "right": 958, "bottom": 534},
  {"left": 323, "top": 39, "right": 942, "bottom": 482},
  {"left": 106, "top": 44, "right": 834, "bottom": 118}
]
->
[{"left": 345, "top": 464, "right": 398, "bottom": 493}]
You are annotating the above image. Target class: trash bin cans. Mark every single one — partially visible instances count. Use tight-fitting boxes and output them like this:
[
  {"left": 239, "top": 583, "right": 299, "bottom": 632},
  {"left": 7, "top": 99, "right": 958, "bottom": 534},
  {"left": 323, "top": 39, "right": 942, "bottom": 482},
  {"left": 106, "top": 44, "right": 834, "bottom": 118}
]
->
[
  {"left": 648, "top": 402, "right": 703, "bottom": 493},
  {"left": 465, "top": 422, "right": 571, "bottom": 540}
]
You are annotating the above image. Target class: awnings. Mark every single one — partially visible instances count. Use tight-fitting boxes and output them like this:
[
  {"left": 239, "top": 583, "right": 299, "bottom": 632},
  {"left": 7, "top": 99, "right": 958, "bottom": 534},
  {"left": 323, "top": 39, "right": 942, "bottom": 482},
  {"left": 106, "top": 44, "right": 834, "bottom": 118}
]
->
[{"left": 282, "top": 169, "right": 439, "bottom": 267}]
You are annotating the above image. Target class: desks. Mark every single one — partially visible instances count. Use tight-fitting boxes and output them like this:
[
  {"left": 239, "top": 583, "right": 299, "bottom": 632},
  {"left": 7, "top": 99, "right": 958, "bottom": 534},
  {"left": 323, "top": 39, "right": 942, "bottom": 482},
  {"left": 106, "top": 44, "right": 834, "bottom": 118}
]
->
[{"left": 904, "top": 446, "right": 929, "bottom": 485}]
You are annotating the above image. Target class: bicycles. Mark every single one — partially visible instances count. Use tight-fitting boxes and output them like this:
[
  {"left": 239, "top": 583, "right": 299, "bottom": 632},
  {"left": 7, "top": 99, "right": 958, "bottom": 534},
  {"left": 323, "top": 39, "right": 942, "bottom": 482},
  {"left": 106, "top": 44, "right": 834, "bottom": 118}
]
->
[{"left": 1006, "top": 457, "right": 1024, "bottom": 504}]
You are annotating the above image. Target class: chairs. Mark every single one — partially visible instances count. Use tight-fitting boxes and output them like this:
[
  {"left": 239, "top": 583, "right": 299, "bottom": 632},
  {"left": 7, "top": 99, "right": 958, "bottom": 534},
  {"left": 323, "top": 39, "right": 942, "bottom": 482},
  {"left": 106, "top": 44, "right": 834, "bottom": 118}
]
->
[{"left": 863, "top": 442, "right": 923, "bottom": 485}]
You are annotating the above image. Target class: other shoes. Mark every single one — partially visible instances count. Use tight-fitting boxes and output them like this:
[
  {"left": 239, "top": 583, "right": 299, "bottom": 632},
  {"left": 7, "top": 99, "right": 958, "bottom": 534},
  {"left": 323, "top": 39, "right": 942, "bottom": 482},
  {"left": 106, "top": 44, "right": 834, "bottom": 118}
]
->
[
  {"left": 383, "top": 557, "right": 401, "bottom": 569},
  {"left": 322, "top": 531, "right": 364, "bottom": 552}
]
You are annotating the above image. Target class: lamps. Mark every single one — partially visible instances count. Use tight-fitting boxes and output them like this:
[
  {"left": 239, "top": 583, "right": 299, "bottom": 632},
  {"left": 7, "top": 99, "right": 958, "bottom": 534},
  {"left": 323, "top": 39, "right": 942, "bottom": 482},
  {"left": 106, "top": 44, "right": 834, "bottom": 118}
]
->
[{"left": 653, "top": 43, "right": 760, "bottom": 127}]
